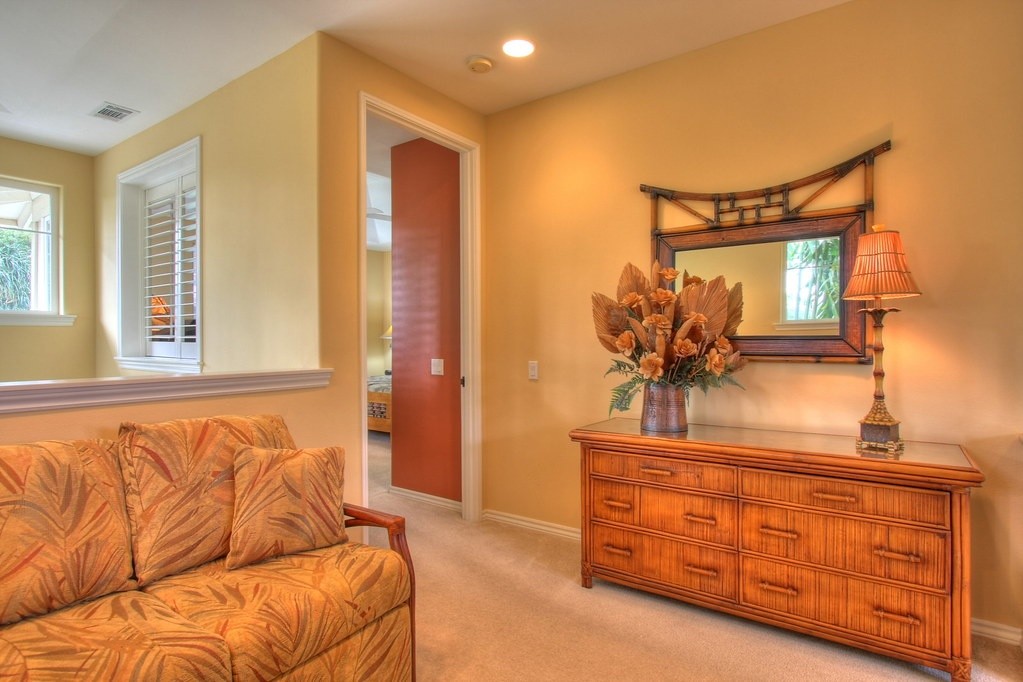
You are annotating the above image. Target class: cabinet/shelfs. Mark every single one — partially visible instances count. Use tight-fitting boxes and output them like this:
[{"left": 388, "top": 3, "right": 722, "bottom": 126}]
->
[{"left": 567, "top": 415, "right": 985, "bottom": 682}]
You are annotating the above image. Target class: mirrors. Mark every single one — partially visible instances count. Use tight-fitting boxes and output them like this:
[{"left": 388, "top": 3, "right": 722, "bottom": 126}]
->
[{"left": 639, "top": 140, "right": 894, "bottom": 366}]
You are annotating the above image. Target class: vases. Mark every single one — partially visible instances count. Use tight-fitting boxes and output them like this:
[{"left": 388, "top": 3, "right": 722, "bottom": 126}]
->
[{"left": 640, "top": 381, "right": 690, "bottom": 433}]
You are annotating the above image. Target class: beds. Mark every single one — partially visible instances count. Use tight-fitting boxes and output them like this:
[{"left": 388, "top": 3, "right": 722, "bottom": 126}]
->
[{"left": 366, "top": 375, "right": 392, "bottom": 449}]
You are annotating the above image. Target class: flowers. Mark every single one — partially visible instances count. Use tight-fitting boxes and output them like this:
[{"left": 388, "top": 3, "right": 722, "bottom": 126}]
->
[
  {"left": 590, "top": 259, "right": 747, "bottom": 420},
  {"left": 682, "top": 268, "right": 744, "bottom": 337}
]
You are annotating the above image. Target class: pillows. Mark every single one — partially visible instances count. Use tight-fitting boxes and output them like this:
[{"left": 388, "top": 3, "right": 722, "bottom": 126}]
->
[
  {"left": 117, "top": 416, "right": 297, "bottom": 591},
  {"left": 226, "top": 438, "right": 351, "bottom": 571},
  {"left": 0, "top": 437, "right": 136, "bottom": 626}
]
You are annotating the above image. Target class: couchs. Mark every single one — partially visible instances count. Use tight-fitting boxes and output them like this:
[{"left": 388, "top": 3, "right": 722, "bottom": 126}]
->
[{"left": 0, "top": 413, "right": 419, "bottom": 682}]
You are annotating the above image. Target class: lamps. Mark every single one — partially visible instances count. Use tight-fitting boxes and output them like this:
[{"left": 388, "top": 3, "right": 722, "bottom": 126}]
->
[
  {"left": 378, "top": 325, "right": 392, "bottom": 375},
  {"left": 840, "top": 222, "right": 923, "bottom": 456},
  {"left": 151, "top": 294, "right": 174, "bottom": 332}
]
